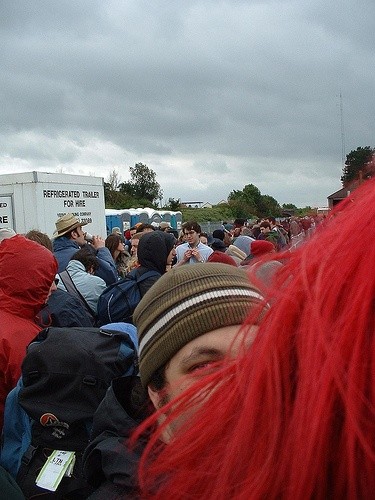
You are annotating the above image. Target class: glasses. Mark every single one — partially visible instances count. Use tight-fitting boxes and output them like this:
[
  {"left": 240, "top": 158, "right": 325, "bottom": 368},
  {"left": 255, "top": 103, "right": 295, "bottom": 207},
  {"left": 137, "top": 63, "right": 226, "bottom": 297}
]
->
[{"left": 185, "top": 231, "right": 196, "bottom": 235}]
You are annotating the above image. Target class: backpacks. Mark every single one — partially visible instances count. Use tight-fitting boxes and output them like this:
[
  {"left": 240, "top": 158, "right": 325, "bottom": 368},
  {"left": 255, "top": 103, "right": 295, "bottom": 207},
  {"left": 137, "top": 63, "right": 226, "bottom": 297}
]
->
[
  {"left": 16, "top": 328, "right": 139, "bottom": 500},
  {"left": 97, "top": 269, "right": 161, "bottom": 328}
]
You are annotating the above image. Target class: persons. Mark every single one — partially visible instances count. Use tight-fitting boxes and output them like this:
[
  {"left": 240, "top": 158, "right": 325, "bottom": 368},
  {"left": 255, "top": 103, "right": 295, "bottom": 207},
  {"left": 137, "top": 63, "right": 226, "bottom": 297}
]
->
[
  {"left": 0, "top": 234, "right": 57, "bottom": 393},
  {"left": 176, "top": 222, "right": 214, "bottom": 266},
  {"left": 1, "top": 217, "right": 351, "bottom": 477},
  {"left": 74, "top": 264, "right": 271, "bottom": 499},
  {"left": 53, "top": 214, "right": 118, "bottom": 283}
]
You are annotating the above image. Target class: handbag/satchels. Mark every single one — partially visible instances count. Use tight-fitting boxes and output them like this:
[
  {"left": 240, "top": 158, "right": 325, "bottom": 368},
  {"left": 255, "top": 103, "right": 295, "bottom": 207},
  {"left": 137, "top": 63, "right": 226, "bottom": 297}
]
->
[{"left": 34, "top": 288, "right": 93, "bottom": 327}]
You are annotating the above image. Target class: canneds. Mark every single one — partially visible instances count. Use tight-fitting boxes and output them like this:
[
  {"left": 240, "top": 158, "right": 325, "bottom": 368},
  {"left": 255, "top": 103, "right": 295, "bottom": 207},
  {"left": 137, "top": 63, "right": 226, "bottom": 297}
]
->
[{"left": 84, "top": 232, "right": 94, "bottom": 244}]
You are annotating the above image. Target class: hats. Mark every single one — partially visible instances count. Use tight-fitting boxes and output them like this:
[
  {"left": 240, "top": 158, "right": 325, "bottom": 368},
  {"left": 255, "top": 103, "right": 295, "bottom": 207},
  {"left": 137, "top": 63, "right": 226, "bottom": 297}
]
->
[
  {"left": 133, "top": 263, "right": 271, "bottom": 394},
  {"left": 159, "top": 222, "right": 169, "bottom": 229},
  {"left": 250, "top": 240, "right": 275, "bottom": 256},
  {"left": 52, "top": 213, "right": 88, "bottom": 239}
]
